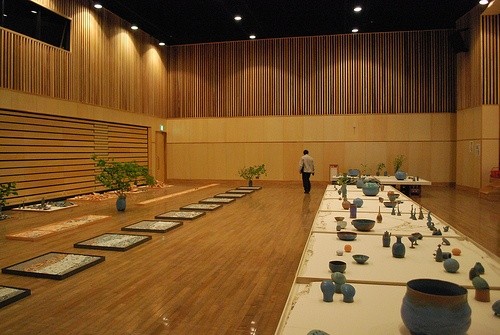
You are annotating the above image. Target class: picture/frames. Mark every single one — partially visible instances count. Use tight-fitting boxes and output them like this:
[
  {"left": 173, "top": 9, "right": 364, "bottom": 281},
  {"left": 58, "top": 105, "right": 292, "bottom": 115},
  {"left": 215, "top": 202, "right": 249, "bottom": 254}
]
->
[
  {"left": 73, "top": 232, "right": 153, "bottom": 252},
  {"left": 225, "top": 189, "right": 255, "bottom": 194},
  {"left": 199, "top": 197, "right": 236, "bottom": 204},
  {"left": 0, "top": 285, "right": 31, "bottom": 309},
  {"left": 154, "top": 210, "right": 206, "bottom": 221},
  {"left": 214, "top": 193, "right": 246, "bottom": 198},
  {"left": 180, "top": 203, "right": 224, "bottom": 211},
  {"left": 121, "top": 220, "right": 184, "bottom": 234},
  {"left": 236, "top": 186, "right": 263, "bottom": 190},
  {"left": 2, "top": 251, "right": 106, "bottom": 281}
]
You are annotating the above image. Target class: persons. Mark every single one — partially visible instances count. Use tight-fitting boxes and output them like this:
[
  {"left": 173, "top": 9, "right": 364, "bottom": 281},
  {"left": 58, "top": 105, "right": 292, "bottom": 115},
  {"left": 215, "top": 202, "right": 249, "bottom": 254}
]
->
[{"left": 300, "top": 149, "right": 315, "bottom": 193}]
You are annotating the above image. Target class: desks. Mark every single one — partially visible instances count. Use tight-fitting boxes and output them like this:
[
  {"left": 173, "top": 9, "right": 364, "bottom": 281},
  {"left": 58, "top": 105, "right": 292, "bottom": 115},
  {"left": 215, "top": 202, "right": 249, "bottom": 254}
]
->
[{"left": 275, "top": 176, "right": 500, "bottom": 335}]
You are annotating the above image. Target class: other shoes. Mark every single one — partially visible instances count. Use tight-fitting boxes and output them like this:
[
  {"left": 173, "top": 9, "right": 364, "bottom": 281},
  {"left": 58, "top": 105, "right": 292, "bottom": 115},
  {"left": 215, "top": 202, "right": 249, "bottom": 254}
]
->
[{"left": 304, "top": 190, "right": 309, "bottom": 193}]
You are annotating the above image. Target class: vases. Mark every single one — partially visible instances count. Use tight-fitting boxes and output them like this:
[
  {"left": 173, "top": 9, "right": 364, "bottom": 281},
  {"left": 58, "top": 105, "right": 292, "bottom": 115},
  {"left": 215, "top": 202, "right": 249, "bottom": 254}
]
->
[{"left": 392, "top": 234, "right": 406, "bottom": 259}]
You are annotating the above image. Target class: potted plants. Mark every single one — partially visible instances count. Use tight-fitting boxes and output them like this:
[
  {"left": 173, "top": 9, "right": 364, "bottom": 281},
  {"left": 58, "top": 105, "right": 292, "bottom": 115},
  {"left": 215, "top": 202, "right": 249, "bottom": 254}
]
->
[
  {"left": 337, "top": 173, "right": 350, "bottom": 197},
  {"left": 375, "top": 162, "right": 386, "bottom": 176},
  {"left": 238, "top": 163, "right": 267, "bottom": 186},
  {"left": 90, "top": 153, "right": 159, "bottom": 212}
]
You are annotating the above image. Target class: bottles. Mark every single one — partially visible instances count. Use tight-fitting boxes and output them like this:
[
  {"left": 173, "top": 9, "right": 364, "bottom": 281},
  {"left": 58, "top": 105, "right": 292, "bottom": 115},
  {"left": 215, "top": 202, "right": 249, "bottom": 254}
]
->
[{"left": 392, "top": 235, "right": 405, "bottom": 258}]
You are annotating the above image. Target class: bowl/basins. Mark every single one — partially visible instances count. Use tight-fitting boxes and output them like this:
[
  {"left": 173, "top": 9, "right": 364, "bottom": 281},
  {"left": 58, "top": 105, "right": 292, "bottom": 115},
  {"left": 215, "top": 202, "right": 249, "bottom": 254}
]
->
[
  {"left": 334, "top": 217, "right": 344, "bottom": 221},
  {"left": 337, "top": 232, "right": 357, "bottom": 241},
  {"left": 329, "top": 261, "right": 346, "bottom": 273},
  {"left": 394, "top": 194, "right": 400, "bottom": 198},
  {"left": 400, "top": 277, "right": 472, "bottom": 335},
  {"left": 395, "top": 169, "right": 405, "bottom": 180},
  {"left": 384, "top": 202, "right": 397, "bottom": 207},
  {"left": 351, "top": 219, "right": 375, "bottom": 232},
  {"left": 363, "top": 182, "right": 379, "bottom": 196},
  {"left": 352, "top": 254, "right": 369, "bottom": 264}
]
